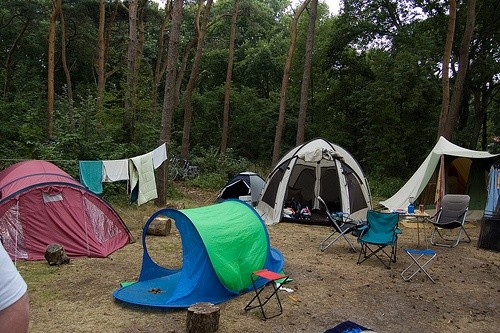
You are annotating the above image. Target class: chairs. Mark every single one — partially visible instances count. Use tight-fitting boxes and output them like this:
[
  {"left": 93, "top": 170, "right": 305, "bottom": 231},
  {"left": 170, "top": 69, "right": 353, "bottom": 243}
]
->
[
  {"left": 315, "top": 196, "right": 357, "bottom": 252},
  {"left": 357, "top": 209, "right": 402, "bottom": 270},
  {"left": 425, "top": 194, "right": 472, "bottom": 248}
]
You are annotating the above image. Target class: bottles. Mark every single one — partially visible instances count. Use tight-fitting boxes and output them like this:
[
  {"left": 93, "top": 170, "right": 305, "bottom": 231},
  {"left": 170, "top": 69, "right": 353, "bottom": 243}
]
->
[
  {"left": 419, "top": 203, "right": 424, "bottom": 214},
  {"left": 408, "top": 204, "right": 414, "bottom": 213}
]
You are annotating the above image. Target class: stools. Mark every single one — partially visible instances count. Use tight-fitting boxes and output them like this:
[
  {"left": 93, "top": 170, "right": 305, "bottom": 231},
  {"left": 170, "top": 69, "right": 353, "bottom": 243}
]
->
[
  {"left": 401, "top": 248, "right": 438, "bottom": 284},
  {"left": 244, "top": 269, "right": 289, "bottom": 319}
]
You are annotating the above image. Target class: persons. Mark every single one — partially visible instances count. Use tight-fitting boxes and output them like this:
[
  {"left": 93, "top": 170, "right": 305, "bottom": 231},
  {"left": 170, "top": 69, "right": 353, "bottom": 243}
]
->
[{"left": 0, "top": 240, "right": 30, "bottom": 333}]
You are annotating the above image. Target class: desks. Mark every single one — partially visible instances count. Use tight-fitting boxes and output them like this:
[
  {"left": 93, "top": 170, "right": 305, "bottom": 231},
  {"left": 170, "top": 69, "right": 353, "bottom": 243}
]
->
[{"left": 379, "top": 210, "right": 430, "bottom": 246}]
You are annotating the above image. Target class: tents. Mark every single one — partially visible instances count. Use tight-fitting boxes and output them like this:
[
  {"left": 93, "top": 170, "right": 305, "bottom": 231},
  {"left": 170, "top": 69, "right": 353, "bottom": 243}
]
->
[
  {"left": 0, "top": 160, "right": 136, "bottom": 262},
  {"left": 252, "top": 137, "right": 374, "bottom": 226},
  {"left": 214, "top": 172, "right": 267, "bottom": 208},
  {"left": 113, "top": 198, "right": 292, "bottom": 307},
  {"left": 378, "top": 136, "right": 500, "bottom": 212}
]
